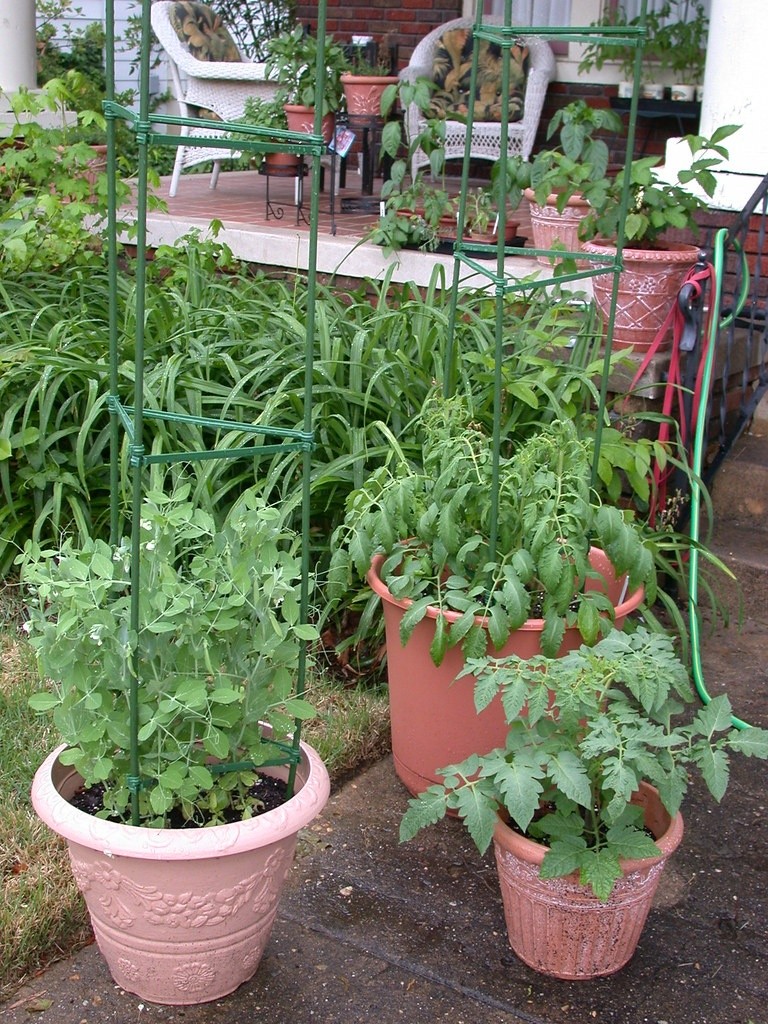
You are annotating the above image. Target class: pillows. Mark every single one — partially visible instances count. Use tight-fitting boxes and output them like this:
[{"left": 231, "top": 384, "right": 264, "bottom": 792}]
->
[
  {"left": 421, "top": 26, "right": 530, "bottom": 123},
  {"left": 166, "top": 0, "right": 246, "bottom": 120}
]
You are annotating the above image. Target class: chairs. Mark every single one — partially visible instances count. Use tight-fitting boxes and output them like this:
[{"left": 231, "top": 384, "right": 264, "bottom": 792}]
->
[
  {"left": 149, "top": 0, "right": 341, "bottom": 205},
  {"left": 399, "top": 15, "right": 558, "bottom": 193}
]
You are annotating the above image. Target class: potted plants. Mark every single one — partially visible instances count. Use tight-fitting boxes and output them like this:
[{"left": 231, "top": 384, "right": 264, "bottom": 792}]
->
[
  {"left": 577, "top": 123, "right": 743, "bottom": 354},
  {"left": 221, "top": 94, "right": 300, "bottom": 175},
  {"left": 377, "top": 77, "right": 440, "bottom": 223},
  {"left": 397, "top": 623, "right": 767, "bottom": 980},
  {"left": 44, "top": 126, "right": 107, "bottom": 204},
  {"left": 630, "top": 9, "right": 673, "bottom": 100},
  {"left": 479, "top": 187, "right": 521, "bottom": 242},
  {"left": 262, "top": 22, "right": 346, "bottom": 144},
  {"left": 325, "top": 389, "right": 661, "bottom": 820},
  {"left": 692, "top": 16, "right": 710, "bottom": 102},
  {"left": 661, "top": 0, "right": 704, "bottom": 101},
  {"left": 338, "top": 38, "right": 400, "bottom": 124},
  {"left": 577, "top": 6, "right": 642, "bottom": 98},
  {"left": 489, "top": 99, "right": 623, "bottom": 272},
  {"left": 406, "top": 110, "right": 476, "bottom": 235},
  {"left": 13, "top": 483, "right": 332, "bottom": 1006}
]
